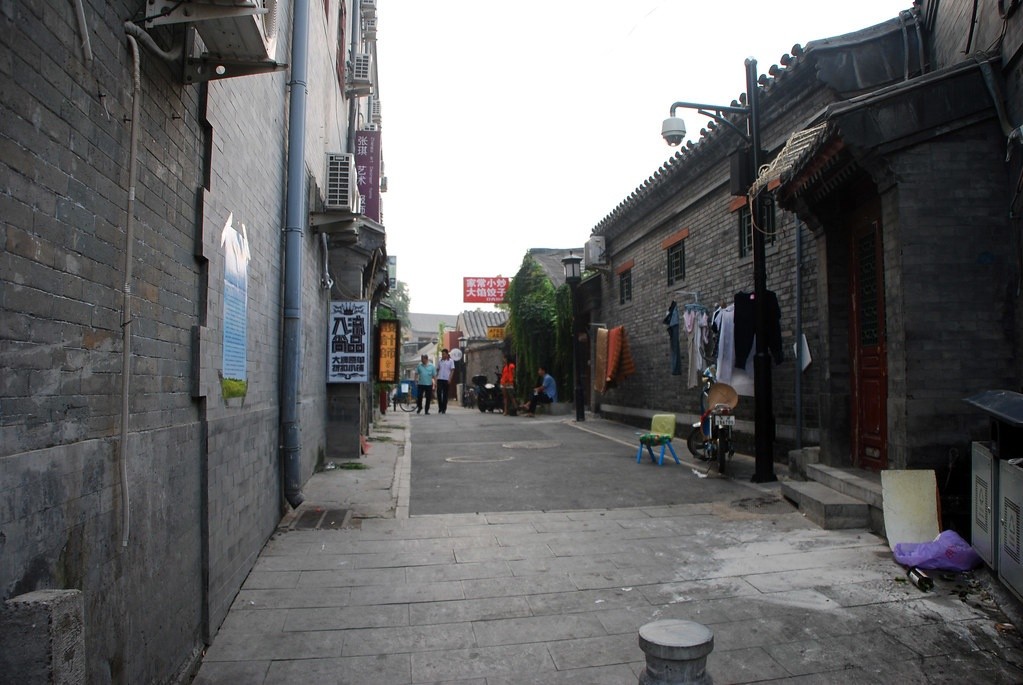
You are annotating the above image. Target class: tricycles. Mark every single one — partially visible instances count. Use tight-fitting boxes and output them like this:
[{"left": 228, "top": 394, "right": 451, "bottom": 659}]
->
[{"left": 398, "top": 374, "right": 419, "bottom": 413}]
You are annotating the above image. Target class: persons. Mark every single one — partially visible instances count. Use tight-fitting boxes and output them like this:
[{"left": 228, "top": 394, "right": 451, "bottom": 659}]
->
[
  {"left": 518, "top": 367, "right": 557, "bottom": 417},
  {"left": 436, "top": 349, "right": 455, "bottom": 414},
  {"left": 414, "top": 354, "right": 436, "bottom": 415},
  {"left": 499, "top": 354, "right": 518, "bottom": 417}
]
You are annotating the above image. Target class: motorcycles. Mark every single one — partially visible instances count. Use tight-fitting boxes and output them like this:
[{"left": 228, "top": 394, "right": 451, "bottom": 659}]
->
[
  {"left": 472, "top": 364, "right": 512, "bottom": 414},
  {"left": 686, "top": 363, "right": 739, "bottom": 477}
]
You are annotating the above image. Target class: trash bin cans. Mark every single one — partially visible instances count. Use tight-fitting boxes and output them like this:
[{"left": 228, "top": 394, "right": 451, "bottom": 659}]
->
[{"left": 961, "top": 388, "right": 1023, "bottom": 604}]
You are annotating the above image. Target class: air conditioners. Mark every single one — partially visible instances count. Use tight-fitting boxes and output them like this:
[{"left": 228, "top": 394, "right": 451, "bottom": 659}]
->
[
  {"left": 324, "top": 151, "right": 362, "bottom": 214},
  {"left": 146, "top": 0, "right": 284, "bottom": 65},
  {"left": 584, "top": 235, "right": 607, "bottom": 268},
  {"left": 352, "top": 52, "right": 372, "bottom": 86},
  {"left": 372, "top": 100, "right": 381, "bottom": 117}
]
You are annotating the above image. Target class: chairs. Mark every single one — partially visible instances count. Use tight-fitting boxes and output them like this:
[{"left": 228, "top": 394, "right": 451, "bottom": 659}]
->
[{"left": 636, "top": 412, "right": 682, "bottom": 468}]
